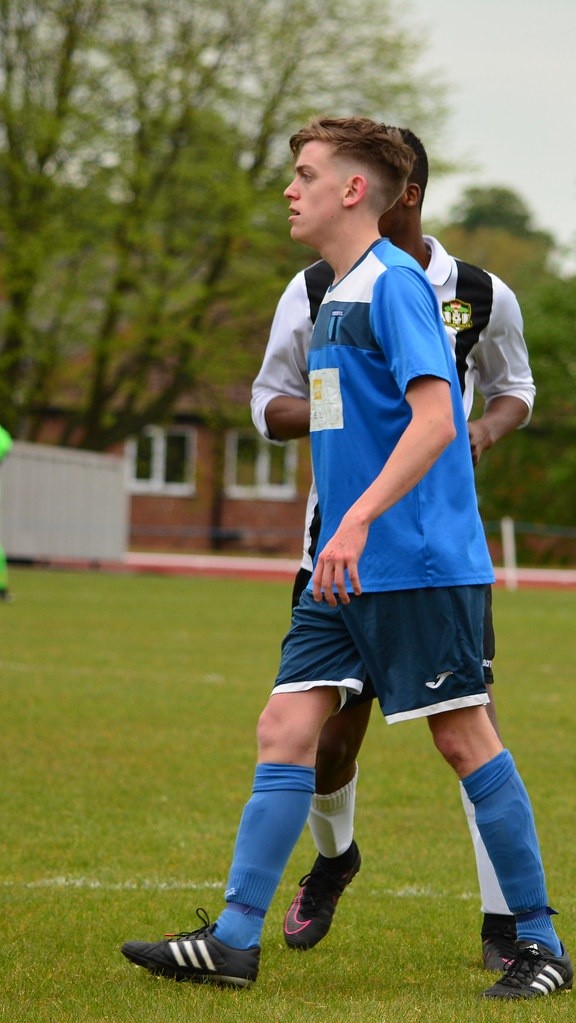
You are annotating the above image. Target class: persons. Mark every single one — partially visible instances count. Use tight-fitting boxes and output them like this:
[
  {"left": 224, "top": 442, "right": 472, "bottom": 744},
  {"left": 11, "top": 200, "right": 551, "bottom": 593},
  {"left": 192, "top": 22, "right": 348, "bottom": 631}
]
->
[
  {"left": 251, "top": 126, "right": 534, "bottom": 974},
  {"left": 120, "top": 113, "right": 573, "bottom": 1000}
]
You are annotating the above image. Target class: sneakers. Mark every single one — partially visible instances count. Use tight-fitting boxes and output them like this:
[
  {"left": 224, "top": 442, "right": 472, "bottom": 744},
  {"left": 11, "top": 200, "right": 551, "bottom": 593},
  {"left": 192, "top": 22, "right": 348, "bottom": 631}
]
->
[
  {"left": 479, "top": 944, "right": 572, "bottom": 999},
  {"left": 482, "top": 914, "right": 517, "bottom": 972},
  {"left": 120, "top": 921, "right": 261, "bottom": 989},
  {"left": 285, "top": 839, "right": 362, "bottom": 948}
]
